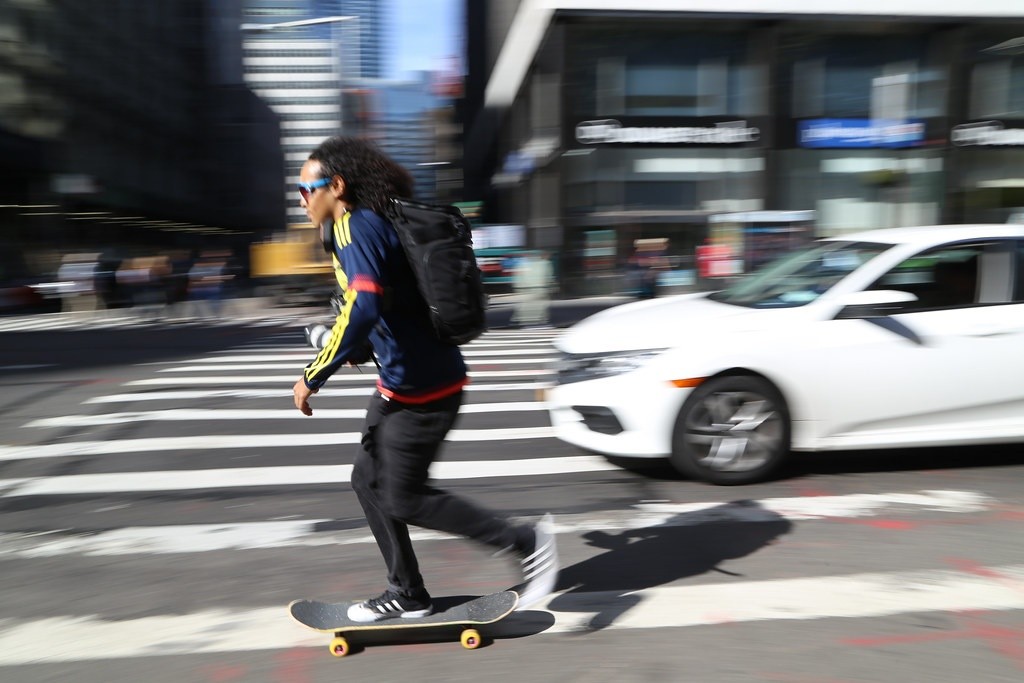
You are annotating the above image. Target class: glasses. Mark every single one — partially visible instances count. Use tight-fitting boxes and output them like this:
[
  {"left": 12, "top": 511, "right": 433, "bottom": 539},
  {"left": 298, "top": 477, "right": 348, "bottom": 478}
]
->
[{"left": 296, "top": 178, "right": 332, "bottom": 201}]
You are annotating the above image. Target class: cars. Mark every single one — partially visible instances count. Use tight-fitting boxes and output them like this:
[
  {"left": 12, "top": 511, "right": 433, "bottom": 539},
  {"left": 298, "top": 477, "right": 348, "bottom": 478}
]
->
[{"left": 543, "top": 222, "right": 1024, "bottom": 486}]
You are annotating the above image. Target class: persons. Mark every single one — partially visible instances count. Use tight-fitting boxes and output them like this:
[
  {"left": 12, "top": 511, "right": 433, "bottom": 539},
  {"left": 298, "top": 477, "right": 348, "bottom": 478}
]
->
[{"left": 292, "top": 132, "right": 554, "bottom": 622}]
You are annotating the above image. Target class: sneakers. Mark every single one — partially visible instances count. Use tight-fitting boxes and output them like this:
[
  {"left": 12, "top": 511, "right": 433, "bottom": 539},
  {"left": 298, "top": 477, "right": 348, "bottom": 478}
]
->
[
  {"left": 517, "top": 522, "right": 555, "bottom": 610},
  {"left": 345, "top": 586, "right": 432, "bottom": 621}
]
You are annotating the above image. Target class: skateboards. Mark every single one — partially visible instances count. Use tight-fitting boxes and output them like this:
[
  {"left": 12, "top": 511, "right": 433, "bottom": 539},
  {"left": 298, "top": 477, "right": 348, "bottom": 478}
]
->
[{"left": 288, "top": 590, "right": 518, "bottom": 657}]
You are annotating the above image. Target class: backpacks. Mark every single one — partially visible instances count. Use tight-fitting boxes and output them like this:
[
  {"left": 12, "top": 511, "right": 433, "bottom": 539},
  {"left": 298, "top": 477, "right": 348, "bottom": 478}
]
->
[{"left": 385, "top": 198, "right": 488, "bottom": 347}]
